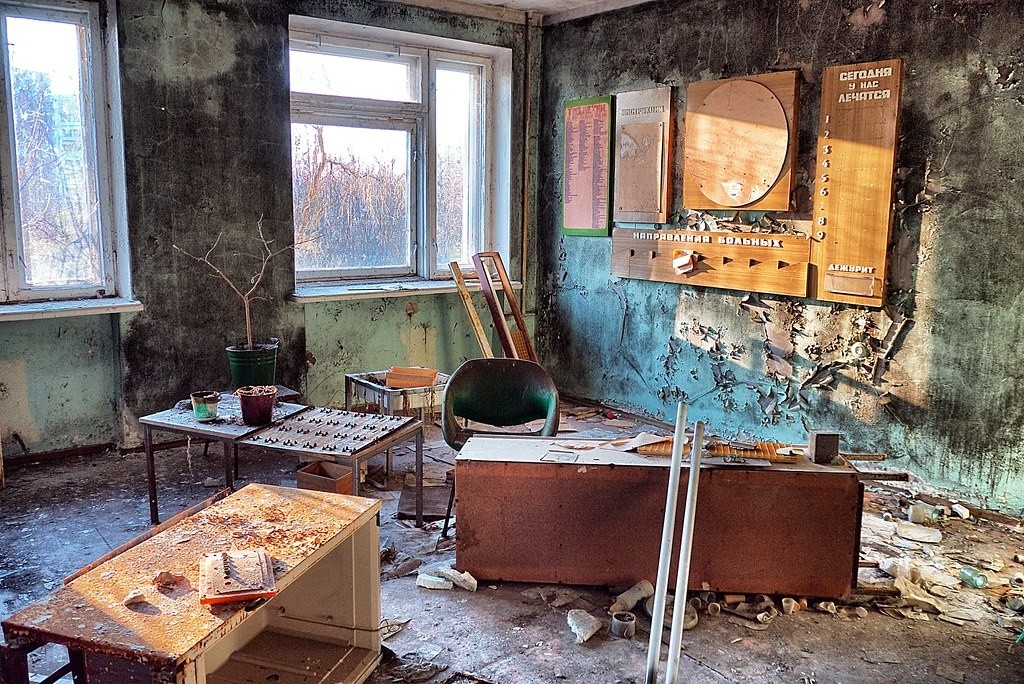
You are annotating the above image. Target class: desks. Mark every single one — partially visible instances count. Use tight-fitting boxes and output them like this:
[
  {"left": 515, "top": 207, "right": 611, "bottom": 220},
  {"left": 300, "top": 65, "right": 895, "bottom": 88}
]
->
[
  {"left": 204, "top": 385, "right": 301, "bottom": 480},
  {"left": 138, "top": 393, "right": 425, "bottom": 529}
]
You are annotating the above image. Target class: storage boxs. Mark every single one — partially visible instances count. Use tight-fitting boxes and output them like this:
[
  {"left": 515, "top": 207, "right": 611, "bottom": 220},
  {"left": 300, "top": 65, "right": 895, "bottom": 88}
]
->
[{"left": 297, "top": 461, "right": 354, "bottom": 496}]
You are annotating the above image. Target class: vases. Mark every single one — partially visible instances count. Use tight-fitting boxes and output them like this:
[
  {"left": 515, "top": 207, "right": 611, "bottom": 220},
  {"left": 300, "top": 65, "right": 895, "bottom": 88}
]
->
[
  {"left": 190, "top": 391, "right": 220, "bottom": 422},
  {"left": 226, "top": 343, "right": 279, "bottom": 389},
  {"left": 237, "top": 386, "right": 276, "bottom": 424}
]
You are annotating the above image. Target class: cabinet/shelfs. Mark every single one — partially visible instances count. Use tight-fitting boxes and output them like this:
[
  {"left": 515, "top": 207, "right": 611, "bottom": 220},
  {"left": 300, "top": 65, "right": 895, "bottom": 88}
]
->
[{"left": 0, "top": 482, "right": 383, "bottom": 684}]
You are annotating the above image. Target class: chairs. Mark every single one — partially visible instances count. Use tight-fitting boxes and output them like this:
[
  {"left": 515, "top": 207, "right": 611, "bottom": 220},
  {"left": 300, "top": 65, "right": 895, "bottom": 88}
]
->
[{"left": 441, "top": 358, "right": 560, "bottom": 537}]
